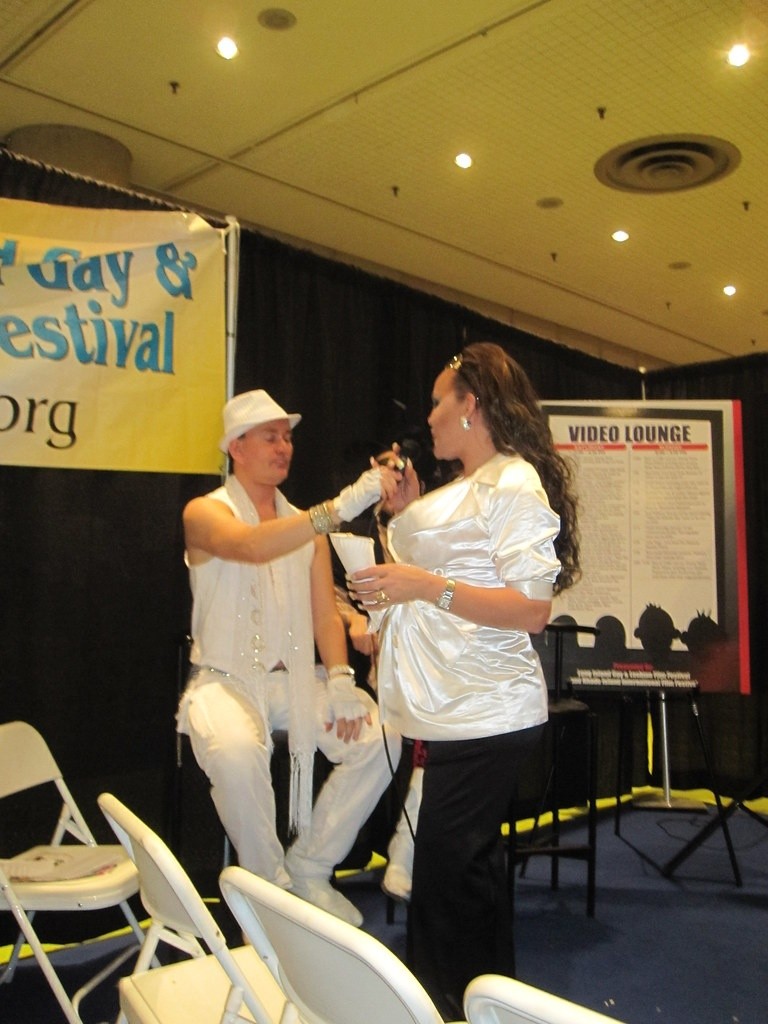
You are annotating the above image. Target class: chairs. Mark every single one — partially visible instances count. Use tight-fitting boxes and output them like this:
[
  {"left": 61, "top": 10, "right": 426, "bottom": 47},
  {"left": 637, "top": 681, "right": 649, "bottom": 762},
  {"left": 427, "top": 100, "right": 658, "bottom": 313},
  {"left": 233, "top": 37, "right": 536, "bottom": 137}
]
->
[
  {"left": 218, "top": 863, "right": 444, "bottom": 1024},
  {"left": 98, "top": 792, "right": 303, "bottom": 1024},
  {"left": 0, "top": 722, "right": 163, "bottom": 1024},
  {"left": 462, "top": 972, "right": 626, "bottom": 1024}
]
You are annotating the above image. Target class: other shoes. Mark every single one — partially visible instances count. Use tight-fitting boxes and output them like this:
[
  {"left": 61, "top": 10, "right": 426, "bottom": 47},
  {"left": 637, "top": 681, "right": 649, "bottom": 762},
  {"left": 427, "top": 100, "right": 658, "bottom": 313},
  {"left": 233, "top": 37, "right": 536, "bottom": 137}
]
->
[{"left": 285, "top": 871, "right": 363, "bottom": 927}]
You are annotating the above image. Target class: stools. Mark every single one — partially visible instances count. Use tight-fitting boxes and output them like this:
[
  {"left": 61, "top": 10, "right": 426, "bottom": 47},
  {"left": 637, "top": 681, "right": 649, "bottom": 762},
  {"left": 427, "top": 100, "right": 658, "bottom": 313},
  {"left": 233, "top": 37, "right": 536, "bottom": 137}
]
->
[{"left": 151, "top": 624, "right": 602, "bottom": 955}]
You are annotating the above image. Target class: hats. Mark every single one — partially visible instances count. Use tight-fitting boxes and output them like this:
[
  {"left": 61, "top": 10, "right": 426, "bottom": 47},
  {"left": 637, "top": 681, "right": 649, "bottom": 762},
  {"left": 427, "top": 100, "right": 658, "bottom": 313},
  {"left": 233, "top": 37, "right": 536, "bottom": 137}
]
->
[{"left": 218, "top": 389, "right": 301, "bottom": 455}]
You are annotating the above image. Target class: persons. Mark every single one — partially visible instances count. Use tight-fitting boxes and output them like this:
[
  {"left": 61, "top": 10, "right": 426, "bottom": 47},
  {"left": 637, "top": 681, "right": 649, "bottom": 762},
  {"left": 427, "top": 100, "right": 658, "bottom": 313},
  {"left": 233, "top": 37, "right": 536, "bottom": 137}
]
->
[
  {"left": 334, "top": 586, "right": 428, "bottom": 905},
  {"left": 346, "top": 343, "right": 584, "bottom": 1024},
  {"left": 175, "top": 390, "right": 403, "bottom": 927}
]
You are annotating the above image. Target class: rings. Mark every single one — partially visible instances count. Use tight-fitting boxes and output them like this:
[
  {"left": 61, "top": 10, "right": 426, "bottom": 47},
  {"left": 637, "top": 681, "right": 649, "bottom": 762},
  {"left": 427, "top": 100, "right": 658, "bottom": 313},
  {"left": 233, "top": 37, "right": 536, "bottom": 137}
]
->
[{"left": 376, "top": 590, "right": 389, "bottom": 603}]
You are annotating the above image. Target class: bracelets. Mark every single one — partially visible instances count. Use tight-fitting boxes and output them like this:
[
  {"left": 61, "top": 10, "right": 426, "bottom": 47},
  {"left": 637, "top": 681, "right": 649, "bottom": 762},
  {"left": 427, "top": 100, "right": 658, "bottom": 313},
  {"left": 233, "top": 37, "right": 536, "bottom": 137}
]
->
[
  {"left": 308, "top": 501, "right": 337, "bottom": 534},
  {"left": 327, "top": 664, "right": 355, "bottom": 677}
]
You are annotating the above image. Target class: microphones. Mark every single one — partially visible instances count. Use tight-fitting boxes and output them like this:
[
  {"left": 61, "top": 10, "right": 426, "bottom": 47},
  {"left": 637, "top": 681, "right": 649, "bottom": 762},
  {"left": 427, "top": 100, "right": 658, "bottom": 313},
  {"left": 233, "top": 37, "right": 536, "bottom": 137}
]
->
[{"left": 374, "top": 441, "right": 420, "bottom": 515}]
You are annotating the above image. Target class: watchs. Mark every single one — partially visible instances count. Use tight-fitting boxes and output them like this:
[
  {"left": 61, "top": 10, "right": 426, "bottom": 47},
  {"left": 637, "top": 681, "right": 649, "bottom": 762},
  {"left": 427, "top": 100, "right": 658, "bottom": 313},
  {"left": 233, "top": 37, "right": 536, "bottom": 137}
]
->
[{"left": 434, "top": 578, "right": 456, "bottom": 611}]
